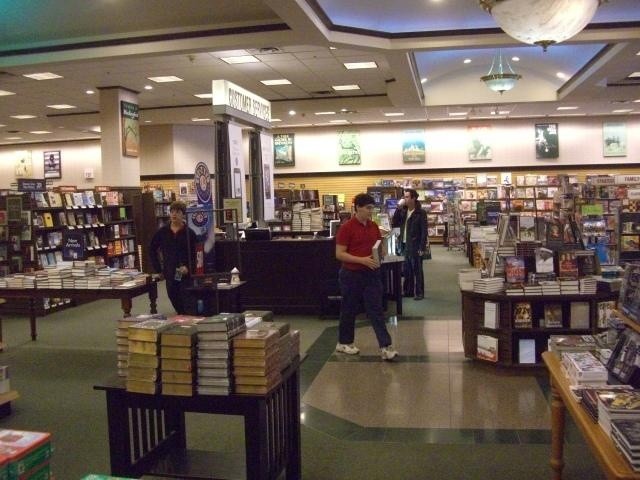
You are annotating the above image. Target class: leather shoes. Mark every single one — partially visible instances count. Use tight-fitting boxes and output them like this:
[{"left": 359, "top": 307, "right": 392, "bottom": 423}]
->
[
  {"left": 414, "top": 296, "right": 425, "bottom": 300},
  {"left": 402, "top": 293, "right": 415, "bottom": 297}
]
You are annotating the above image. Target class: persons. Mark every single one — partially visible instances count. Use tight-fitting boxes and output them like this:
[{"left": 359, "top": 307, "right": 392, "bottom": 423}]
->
[
  {"left": 391, "top": 190, "right": 428, "bottom": 300},
  {"left": 150, "top": 201, "right": 200, "bottom": 316},
  {"left": 335, "top": 193, "right": 400, "bottom": 361}
]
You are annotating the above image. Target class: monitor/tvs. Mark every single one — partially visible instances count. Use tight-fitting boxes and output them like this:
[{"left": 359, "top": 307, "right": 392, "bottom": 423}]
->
[
  {"left": 328, "top": 220, "right": 341, "bottom": 238},
  {"left": 245, "top": 227, "right": 272, "bottom": 241}
]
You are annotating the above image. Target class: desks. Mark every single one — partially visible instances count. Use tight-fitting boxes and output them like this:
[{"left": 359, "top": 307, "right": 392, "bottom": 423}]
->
[
  {"left": 541, "top": 351, "right": 639, "bottom": 479},
  {"left": 93, "top": 354, "right": 309, "bottom": 479},
  {"left": 0, "top": 273, "right": 166, "bottom": 352}
]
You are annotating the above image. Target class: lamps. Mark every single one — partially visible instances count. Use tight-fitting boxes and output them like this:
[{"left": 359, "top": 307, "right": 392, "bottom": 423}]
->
[
  {"left": 480, "top": 47, "right": 521, "bottom": 97},
  {"left": 477, "top": 1, "right": 600, "bottom": 52}
]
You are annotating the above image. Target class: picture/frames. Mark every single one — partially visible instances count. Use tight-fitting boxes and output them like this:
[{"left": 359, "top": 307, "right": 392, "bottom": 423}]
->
[
  {"left": 44, "top": 150, "right": 61, "bottom": 179},
  {"left": 602, "top": 121, "right": 629, "bottom": 157}
]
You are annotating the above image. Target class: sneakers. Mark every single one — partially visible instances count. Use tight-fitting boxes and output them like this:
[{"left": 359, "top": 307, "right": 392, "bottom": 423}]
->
[
  {"left": 381, "top": 347, "right": 398, "bottom": 360},
  {"left": 336, "top": 342, "right": 360, "bottom": 355}
]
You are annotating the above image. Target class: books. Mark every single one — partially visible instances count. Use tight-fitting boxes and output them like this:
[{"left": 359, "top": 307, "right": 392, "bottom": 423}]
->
[
  {"left": 0, "top": 184, "right": 180, "bottom": 310},
  {"left": 0, "top": 425, "right": 142, "bottom": 480},
  {"left": 548, "top": 336, "right": 639, "bottom": 472},
  {"left": 457, "top": 169, "right": 640, "bottom": 363},
  {"left": 0, "top": 364, "right": 11, "bottom": 394},
  {"left": 114, "top": 310, "right": 300, "bottom": 398},
  {"left": 270, "top": 177, "right": 457, "bottom": 268}
]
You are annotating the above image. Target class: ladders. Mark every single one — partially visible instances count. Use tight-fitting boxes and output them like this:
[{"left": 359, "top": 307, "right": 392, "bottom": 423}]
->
[{"left": 447, "top": 198, "right": 462, "bottom": 251}]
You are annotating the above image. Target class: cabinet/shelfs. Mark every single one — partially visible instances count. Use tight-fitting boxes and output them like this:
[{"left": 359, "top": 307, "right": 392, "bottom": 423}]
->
[
  {"left": 275, "top": 189, "right": 319, "bottom": 218},
  {"left": 0, "top": 185, "right": 176, "bottom": 316}
]
